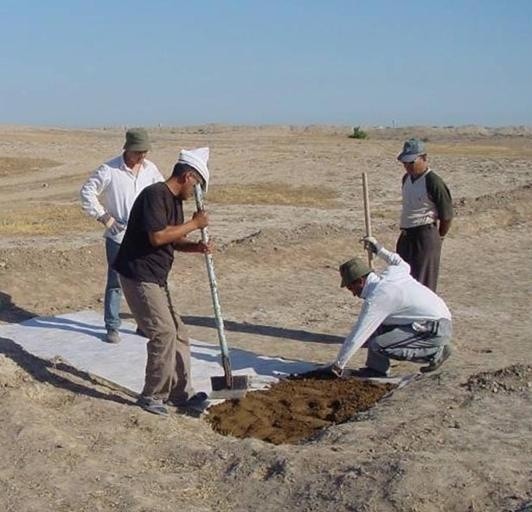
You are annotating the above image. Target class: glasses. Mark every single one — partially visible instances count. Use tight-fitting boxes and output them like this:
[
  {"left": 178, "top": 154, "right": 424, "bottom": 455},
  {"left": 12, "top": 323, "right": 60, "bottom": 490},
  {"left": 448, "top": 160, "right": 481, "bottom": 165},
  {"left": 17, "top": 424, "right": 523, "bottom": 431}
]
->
[{"left": 402, "top": 157, "right": 424, "bottom": 165}]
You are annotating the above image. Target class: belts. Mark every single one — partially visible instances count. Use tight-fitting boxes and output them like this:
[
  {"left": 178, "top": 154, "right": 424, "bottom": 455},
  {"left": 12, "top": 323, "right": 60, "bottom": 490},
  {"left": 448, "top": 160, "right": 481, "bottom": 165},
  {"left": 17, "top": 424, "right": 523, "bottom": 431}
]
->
[{"left": 402, "top": 223, "right": 436, "bottom": 237}]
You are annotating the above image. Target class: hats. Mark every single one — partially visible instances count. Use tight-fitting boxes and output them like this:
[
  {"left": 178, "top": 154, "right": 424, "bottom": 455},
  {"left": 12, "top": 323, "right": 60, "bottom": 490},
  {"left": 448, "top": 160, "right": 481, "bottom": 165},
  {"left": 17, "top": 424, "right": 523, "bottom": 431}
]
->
[
  {"left": 123, "top": 129, "right": 152, "bottom": 152},
  {"left": 340, "top": 257, "right": 375, "bottom": 287},
  {"left": 397, "top": 138, "right": 427, "bottom": 163},
  {"left": 176, "top": 147, "right": 209, "bottom": 193}
]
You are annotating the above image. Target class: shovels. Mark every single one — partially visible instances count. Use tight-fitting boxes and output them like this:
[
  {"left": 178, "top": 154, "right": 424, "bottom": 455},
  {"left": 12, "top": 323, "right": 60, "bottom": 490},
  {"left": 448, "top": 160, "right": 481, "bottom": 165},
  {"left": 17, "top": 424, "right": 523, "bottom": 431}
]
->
[{"left": 192, "top": 182, "right": 248, "bottom": 392}]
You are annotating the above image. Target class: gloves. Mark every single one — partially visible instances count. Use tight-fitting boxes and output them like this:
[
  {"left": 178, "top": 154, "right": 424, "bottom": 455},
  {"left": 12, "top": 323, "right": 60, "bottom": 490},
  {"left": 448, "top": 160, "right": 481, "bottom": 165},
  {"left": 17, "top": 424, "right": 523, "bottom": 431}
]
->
[
  {"left": 290, "top": 364, "right": 344, "bottom": 380},
  {"left": 359, "top": 235, "right": 383, "bottom": 255},
  {"left": 97, "top": 211, "right": 125, "bottom": 236}
]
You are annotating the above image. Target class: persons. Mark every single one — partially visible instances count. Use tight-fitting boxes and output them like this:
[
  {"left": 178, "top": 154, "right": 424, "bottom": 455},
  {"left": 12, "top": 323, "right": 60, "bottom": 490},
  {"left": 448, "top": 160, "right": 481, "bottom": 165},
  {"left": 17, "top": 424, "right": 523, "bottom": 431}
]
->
[
  {"left": 395, "top": 134, "right": 455, "bottom": 297},
  {"left": 109, "top": 145, "right": 216, "bottom": 416},
  {"left": 321, "top": 234, "right": 456, "bottom": 378},
  {"left": 78, "top": 127, "right": 167, "bottom": 345}
]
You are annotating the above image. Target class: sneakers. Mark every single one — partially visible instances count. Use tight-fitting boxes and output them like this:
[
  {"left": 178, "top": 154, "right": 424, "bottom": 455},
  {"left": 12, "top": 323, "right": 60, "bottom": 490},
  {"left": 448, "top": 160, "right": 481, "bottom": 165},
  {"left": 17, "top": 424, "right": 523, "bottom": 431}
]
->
[
  {"left": 167, "top": 392, "right": 207, "bottom": 406},
  {"left": 136, "top": 396, "right": 171, "bottom": 416},
  {"left": 420, "top": 344, "right": 452, "bottom": 373},
  {"left": 106, "top": 328, "right": 118, "bottom": 343},
  {"left": 350, "top": 367, "right": 387, "bottom": 377}
]
